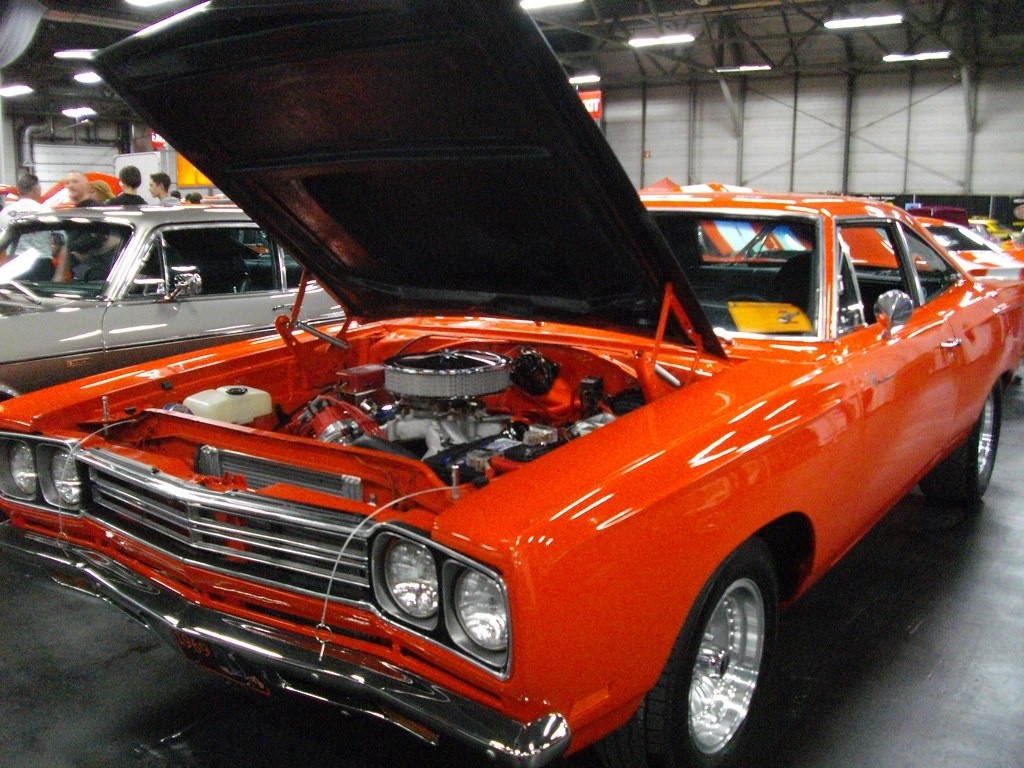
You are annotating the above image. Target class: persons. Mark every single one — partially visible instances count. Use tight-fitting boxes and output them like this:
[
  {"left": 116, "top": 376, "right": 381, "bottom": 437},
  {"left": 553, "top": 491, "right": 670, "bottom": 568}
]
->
[{"left": 0, "top": 165, "right": 228, "bottom": 283}]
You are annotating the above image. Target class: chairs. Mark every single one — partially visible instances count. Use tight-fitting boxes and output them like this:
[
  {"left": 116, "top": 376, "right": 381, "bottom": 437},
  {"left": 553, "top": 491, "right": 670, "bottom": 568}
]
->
[
  {"left": 757, "top": 249, "right": 850, "bottom": 311},
  {"left": 198, "top": 246, "right": 250, "bottom": 297}
]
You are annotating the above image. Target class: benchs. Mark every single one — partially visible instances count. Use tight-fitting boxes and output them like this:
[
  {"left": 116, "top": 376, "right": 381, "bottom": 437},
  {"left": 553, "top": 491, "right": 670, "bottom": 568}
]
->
[
  {"left": 245, "top": 259, "right": 302, "bottom": 292},
  {"left": 690, "top": 265, "right": 943, "bottom": 319}
]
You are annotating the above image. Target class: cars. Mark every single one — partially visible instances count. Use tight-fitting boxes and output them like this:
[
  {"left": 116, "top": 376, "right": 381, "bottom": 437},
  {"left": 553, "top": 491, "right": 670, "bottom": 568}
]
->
[
  {"left": 967, "top": 217, "right": 1018, "bottom": 242},
  {"left": 2, "top": 0, "right": 1023, "bottom": 766},
  {"left": 1, "top": 206, "right": 353, "bottom": 402}
]
